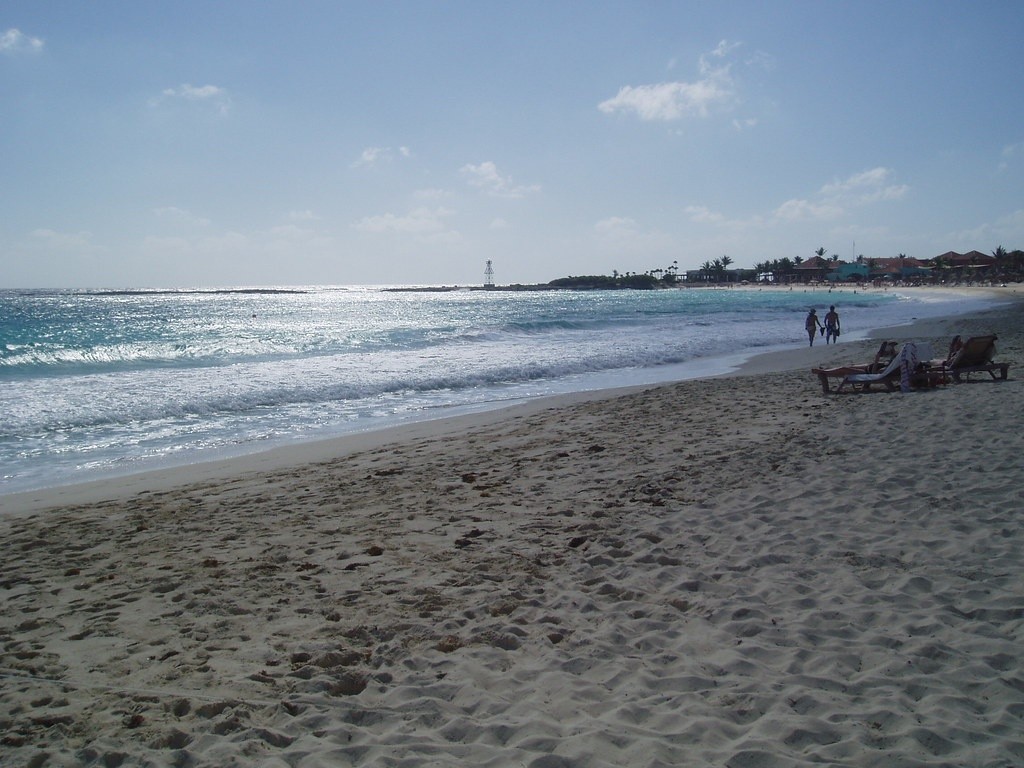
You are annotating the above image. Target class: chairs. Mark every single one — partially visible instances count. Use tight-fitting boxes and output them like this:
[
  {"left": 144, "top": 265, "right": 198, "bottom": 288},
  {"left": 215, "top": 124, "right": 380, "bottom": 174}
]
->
[
  {"left": 835, "top": 342, "right": 930, "bottom": 393},
  {"left": 921, "top": 334, "right": 999, "bottom": 386}
]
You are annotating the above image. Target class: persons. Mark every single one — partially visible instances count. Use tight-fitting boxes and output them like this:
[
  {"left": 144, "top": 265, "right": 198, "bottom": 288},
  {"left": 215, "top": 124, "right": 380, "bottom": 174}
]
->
[
  {"left": 805, "top": 308, "right": 822, "bottom": 347},
  {"left": 811, "top": 341, "right": 899, "bottom": 375},
  {"left": 824, "top": 305, "right": 840, "bottom": 345},
  {"left": 946, "top": 335, "right": 964, "bottom": 365}
]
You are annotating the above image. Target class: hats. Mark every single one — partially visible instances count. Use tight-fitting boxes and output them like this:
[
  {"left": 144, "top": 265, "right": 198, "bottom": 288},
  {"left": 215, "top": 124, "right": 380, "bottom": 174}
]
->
[{"left": 836, "top": 328, "right": 840, "bottom": 337}]
